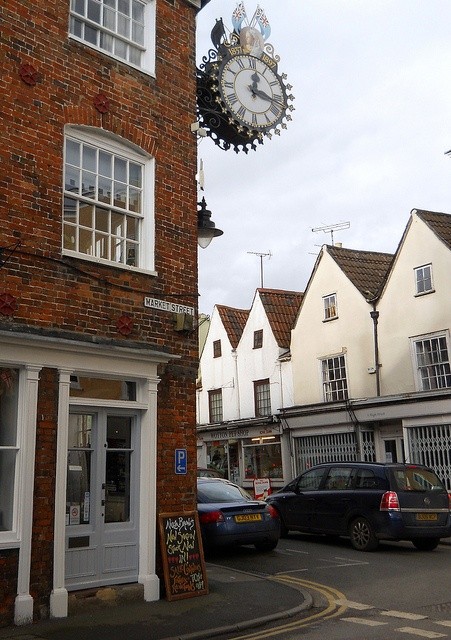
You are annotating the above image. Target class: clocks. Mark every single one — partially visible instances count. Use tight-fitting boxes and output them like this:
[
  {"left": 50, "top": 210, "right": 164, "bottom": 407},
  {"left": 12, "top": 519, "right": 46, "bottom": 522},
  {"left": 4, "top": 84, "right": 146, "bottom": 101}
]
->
[{"left": 198, "top": 0, "right": 295, "bottom": 153}]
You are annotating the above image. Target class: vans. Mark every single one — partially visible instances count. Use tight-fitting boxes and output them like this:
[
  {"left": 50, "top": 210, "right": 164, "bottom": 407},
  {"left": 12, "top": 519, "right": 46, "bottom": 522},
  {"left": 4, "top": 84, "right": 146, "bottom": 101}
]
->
[{"left": 264, "top": 461, "right": 451, "bottom": 551}]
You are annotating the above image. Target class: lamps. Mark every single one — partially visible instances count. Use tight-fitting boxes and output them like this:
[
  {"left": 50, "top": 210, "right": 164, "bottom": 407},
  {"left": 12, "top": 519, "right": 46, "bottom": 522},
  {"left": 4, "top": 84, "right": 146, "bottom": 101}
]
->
[{"left": 196, "top": 196, "right": 224, "bottom": 249}]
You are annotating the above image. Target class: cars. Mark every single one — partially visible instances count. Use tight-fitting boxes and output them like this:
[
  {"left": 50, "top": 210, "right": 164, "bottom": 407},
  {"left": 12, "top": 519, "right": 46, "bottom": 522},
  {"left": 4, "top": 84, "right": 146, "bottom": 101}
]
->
[{"left": 196, "top": 477, "right": 281, "bottom": 557}]
